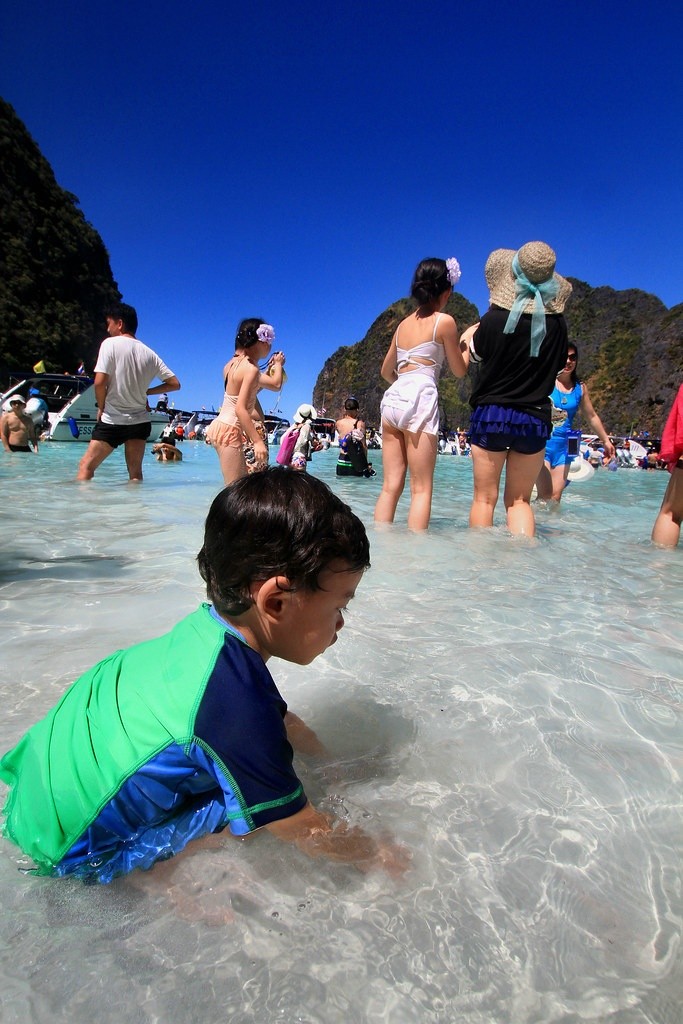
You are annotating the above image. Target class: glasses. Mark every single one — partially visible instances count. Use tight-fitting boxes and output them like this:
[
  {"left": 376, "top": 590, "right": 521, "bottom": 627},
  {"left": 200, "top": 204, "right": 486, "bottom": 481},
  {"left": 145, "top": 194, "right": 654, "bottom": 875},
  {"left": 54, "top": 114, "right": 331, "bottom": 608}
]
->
[{"left": 568, "top": 354, "right": 577, "bottom": 361}]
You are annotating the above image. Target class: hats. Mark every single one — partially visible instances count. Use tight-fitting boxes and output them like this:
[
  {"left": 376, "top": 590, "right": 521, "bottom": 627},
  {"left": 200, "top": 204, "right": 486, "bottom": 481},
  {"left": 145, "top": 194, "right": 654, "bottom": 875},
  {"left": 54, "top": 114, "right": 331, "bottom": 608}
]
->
[
  {"left": 294, "top": 404, "right": 318, "bottom": 423},
  {"left": 10, "top": 395, "right": 26, "bottom": 410},
  {"left": 485, "top": 240, "right": 573, "bottom": 315},
  {"left": 567, "top": 456, "right": 595, "bottom": 482}
]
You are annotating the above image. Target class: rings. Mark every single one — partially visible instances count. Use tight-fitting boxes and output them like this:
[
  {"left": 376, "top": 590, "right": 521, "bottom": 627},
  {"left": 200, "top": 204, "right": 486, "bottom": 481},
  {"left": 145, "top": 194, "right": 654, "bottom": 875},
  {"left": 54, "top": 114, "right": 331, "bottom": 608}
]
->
[{"left": 155, "top": 446, "right": 156, "bottom": 448}]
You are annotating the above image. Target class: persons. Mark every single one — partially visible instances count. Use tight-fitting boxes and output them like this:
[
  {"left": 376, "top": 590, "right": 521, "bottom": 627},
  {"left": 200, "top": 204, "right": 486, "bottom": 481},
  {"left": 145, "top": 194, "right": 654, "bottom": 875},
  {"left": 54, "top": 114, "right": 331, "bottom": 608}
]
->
[
  {"left": 0, "top": 388, "right": 49, "bottom": 455},
  {"left": 146, "top": 394, "right": 203, "bottom": 463},
  {"left": 651, "top": 381, "right": 683, "bottom": 550},
  {"left": 335, "top": 398, "right": 372, "bottom": 477},
  {"left": 533, "top": 343, "right": 616, "bottom": 512},
  {"left": 206, "top": 317, "right": 331, "bottom": 491},
  {"left": 465, "top": 240, "right": 569, "bottom": 544},
  {"left": 444, "top": 431, "right": 466, "bottom": 449},
  {"left": 0, "top": 464, "right": 412, "bottom": 884},
  {"left": 77, "top": 305, "right": 181, "bottom": 484},
  {"left": 583, "top": 430, "right": 668, "bottom": 472},
  {"left": 373, "top": 257, "right": 481, "bottom": 536}
]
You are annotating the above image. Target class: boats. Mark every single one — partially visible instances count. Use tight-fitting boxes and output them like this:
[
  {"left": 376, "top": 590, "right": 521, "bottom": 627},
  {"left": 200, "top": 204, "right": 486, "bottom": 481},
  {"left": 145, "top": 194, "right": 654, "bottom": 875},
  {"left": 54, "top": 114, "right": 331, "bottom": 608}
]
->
[{"left": 0, "top": 375, "right": 170, "bottom": 441}]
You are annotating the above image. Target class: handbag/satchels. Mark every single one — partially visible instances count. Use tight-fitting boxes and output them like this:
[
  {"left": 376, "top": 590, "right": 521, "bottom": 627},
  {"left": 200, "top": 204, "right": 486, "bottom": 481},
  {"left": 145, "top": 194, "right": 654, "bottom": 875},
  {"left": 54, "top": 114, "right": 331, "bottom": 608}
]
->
[{"left": 348, "top": 420, "right": 369, "bottom": 472}]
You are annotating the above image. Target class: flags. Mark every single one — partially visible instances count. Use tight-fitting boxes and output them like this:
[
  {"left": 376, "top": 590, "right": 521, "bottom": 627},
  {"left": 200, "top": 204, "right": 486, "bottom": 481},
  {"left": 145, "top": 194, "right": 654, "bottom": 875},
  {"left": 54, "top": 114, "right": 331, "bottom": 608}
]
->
[
  {"left": 33, "top": 360, "right": 46, "bottom": 374},
  {"left": 78, "top": 361, "right": 86, "bottom": 376}
]
